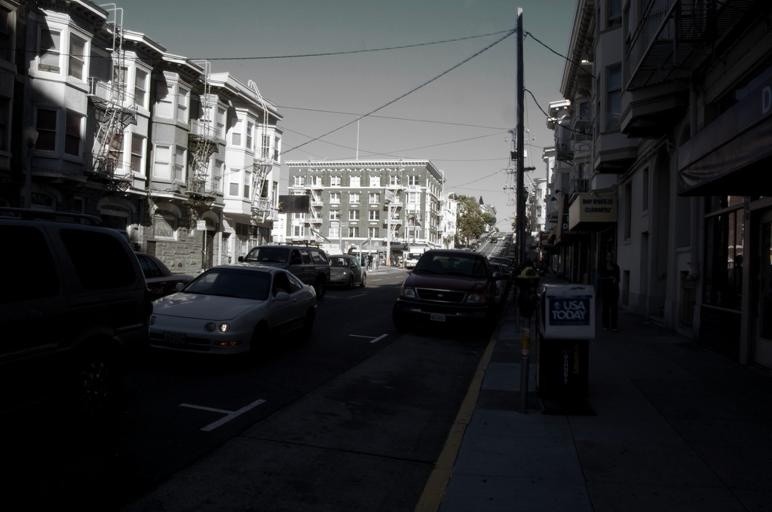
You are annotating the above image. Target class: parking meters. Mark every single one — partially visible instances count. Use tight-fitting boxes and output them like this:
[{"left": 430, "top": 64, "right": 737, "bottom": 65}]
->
[{"left": 517, "top": 266, "right": 541, "bottom": 414}]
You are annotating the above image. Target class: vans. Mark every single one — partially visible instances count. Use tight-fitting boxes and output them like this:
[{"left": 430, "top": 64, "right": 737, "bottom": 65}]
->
[{"left": 1, "top": 206, "right": 153, "bottom": 402}]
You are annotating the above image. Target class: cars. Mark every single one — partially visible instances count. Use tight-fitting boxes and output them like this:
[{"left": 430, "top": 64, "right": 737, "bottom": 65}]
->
[
  {"left": 491, "top": 237, "right": 497, "bottom": 243},
  {"left": 393, "top": 250, "right": 512, "bottom": 329},
  {"left": 136, "top": 245, "right": 368, "bottom": 354}
]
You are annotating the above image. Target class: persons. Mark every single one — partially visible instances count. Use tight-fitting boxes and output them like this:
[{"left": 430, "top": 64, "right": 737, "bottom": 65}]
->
[
  {"left": 354, "top": 251, "right": 406, "bottom": 273},
  {"left": 599, "top": 250, "right": 620, "bottom": 334}
]
